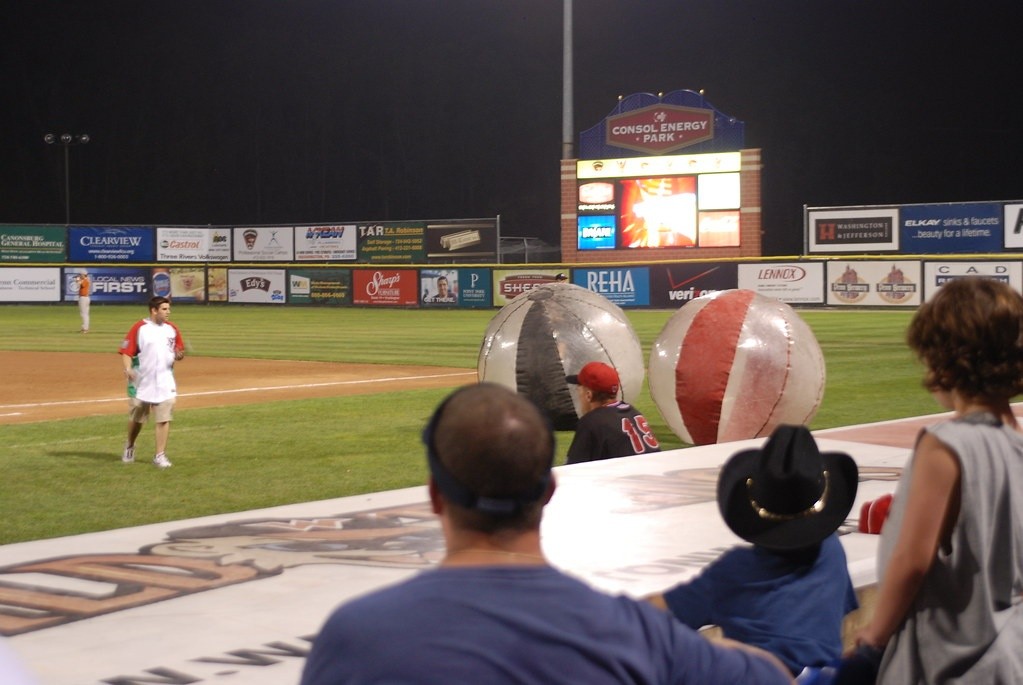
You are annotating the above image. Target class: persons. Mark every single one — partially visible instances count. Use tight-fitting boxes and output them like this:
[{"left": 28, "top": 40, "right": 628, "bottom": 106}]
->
[
  {"left": 853, "top": 274, "right": 1023, "bottom": 685},
  {"left": 564, "top": 361, "right": 662, "bottom": 465},
  {"left": 74, "top": 270, "right": 91, "bottom": 334},
  {"left": 641, "top": 422, "right": 861, "bottom": 685},
  {"left": 299, "top": 381, "right": 797, "bottom": 685},
  {"left": 431, "top": 276, "right": 458, "bottom": 303},
  {"left": 118, "top": 296, "right": 185, "bottom": 467}
]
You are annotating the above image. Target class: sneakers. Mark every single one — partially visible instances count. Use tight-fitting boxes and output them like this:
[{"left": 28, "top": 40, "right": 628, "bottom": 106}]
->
[
  {"left": 122, "top": 447, "right": 136, "bottom": 463},
  {"left": 152, "top": 451, "right": 172, "bottom": 467}
]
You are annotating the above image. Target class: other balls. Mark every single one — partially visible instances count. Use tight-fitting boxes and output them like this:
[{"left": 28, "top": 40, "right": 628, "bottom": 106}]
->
[
  {"left": 651, "top": 290, "right": 827, "bottom": 451},
  {"left": 475, "top": 281, "right": 642, "bottom": 426}
]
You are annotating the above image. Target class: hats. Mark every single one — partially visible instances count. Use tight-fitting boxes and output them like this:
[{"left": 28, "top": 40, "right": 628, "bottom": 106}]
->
[
  {"left": 564, "top": 361, "right": 620, "bottom": 394},
  {"left": 716, "top": 423, "right": 859, "bottom": 549},
  {"left": 79, "top": 268, "right": 88, "bottom": 274}
]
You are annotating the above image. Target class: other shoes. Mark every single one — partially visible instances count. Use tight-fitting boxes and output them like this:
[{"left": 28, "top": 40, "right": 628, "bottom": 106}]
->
[{"left": 80, "top": 329, "right": 88, "bottom": 334}]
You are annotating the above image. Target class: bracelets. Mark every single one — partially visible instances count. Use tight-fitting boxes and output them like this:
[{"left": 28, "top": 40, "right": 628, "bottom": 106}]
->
[{"left": 124, "top": 368, "right": 128, "bottom": 373}]
